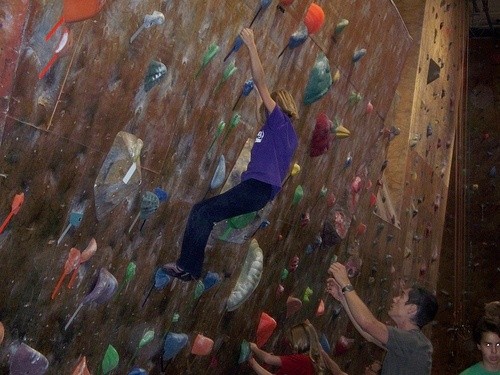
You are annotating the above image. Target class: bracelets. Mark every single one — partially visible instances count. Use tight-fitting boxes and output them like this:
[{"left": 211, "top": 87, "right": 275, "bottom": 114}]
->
[{"left": 342, "top": 283, "right": 354, "bottom": 295}]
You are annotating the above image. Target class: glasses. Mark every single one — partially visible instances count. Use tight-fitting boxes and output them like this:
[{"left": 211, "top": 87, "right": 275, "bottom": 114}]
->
[{"left": 483, "top": 343, "right": 500, "bottom": 350}]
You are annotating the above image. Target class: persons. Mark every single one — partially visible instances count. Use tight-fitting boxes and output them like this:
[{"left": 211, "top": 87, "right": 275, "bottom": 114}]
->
[
  {"left": 459, "top": 322, "right": 500, "bottom": 375},
  {"left": 162, "top": 26, "right": 300, "bottom": 281},
  {"left": 326, "top": 261, "right": 442, "bottom": 375},
  {"left": 246, "top": 320, "right": 327, "bottom": 375},
  {"left": 304, "top": 319, "right": 383, "bottom": 375}
]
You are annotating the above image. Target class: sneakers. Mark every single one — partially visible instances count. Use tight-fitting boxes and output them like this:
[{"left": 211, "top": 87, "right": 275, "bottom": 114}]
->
[{"left": 163, "top": 264, "right": 201, "bottom": 282}]
